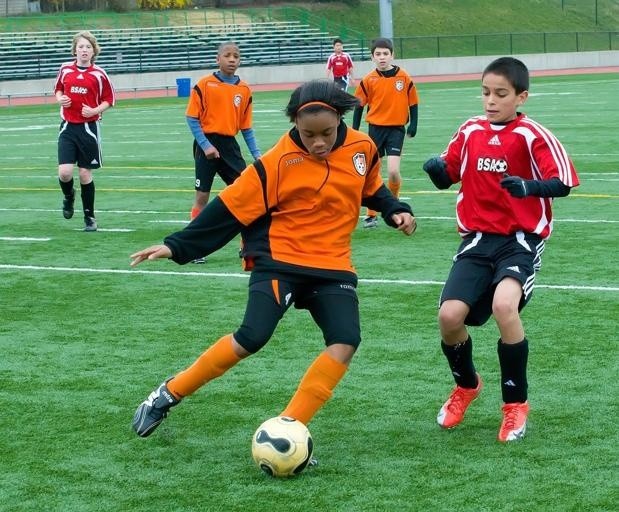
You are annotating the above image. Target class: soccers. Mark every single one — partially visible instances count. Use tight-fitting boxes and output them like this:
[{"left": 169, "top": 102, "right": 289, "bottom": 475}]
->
[{"left": 251, "top": 415, "right": 315, "bottom": 478}]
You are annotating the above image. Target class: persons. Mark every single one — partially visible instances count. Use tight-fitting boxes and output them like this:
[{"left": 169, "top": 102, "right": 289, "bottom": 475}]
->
[
  {"left": 55, "top": 29, "right": 114, "bottom": 232},
  {"left": 353, "top": 37, "right": 419, "bottom": 230},
  {"left": 183, "top": 41, "right": 263, "bottom": 262},
  {"left": 129, "top": 79, "right": 418, "bottom": 467},
  {"left": 421, "top": 55, "right": 580, "bottom": 445},
  {"left": 325, "top": 38, "right": 356, "bottom": 94}
]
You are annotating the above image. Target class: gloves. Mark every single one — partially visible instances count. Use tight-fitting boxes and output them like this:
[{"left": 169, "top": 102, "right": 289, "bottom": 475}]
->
[
  {"left": 422, "top": 157, "right": 448, "bottom": 179},
  {"left": 499, "top": 173, "right": 540, "bottom": 198}
]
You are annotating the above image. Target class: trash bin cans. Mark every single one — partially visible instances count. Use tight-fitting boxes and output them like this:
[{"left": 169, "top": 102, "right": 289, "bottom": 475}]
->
[{"left": 176, "top": 78, "right": 191, "bottom": 97}]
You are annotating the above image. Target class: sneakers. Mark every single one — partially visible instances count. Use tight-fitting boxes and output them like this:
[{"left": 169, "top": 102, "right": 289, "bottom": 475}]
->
[
  {"left": 132, "top": 374, "right": 181, "bottom": 437},
  {"left": 359, "top": 214, "right": 378, "bottom": 228},
  {"left": 437, "top": 372, "right": 481, "bottom": 429},
  {"left": 497, "top": 399, "right": 529, "bottom": 443},
  {"left": 63, "top": 187, "right": 76, "bottom": 219},
  {"left": 83, "top": 215, "right": 98, "bottom": 231}
]
